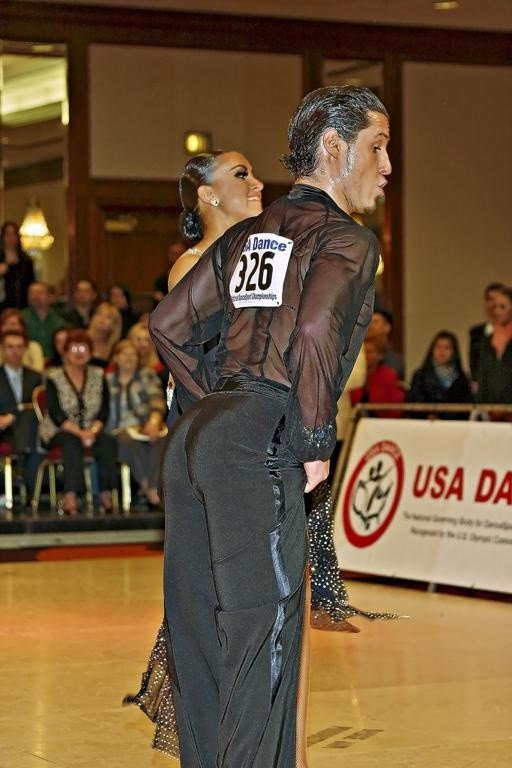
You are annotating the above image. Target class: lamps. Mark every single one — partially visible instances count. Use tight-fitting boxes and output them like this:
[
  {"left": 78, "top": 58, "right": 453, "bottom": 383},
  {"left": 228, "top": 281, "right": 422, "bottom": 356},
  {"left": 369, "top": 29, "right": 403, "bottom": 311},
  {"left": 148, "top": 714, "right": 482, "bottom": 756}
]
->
[{"left": 19, "top": 180, "right": 56, "bottom": 257}]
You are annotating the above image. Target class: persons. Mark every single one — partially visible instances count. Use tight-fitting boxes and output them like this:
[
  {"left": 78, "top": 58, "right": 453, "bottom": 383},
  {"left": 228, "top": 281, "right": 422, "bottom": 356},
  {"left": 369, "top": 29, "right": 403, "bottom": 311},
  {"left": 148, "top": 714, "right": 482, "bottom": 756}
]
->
[
  {"left": 0, "top": 222, "right": 36, "bottom": 311},
  {"left": 148, "top": 82, "right": 394, "bottom": 767},
  {"left": 118, "top": 149, "right": 312, "bottom": 768},
  {"left": 0, "top": 277, "right": 511, "bottom": 514}
]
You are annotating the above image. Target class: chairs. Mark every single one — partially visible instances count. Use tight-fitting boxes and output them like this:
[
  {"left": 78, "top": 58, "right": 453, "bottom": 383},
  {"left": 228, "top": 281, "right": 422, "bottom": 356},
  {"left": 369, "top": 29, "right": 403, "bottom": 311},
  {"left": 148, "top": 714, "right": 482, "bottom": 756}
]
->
[{"left": 2, "top": 381, "right": 137, "bottom": 517}]
